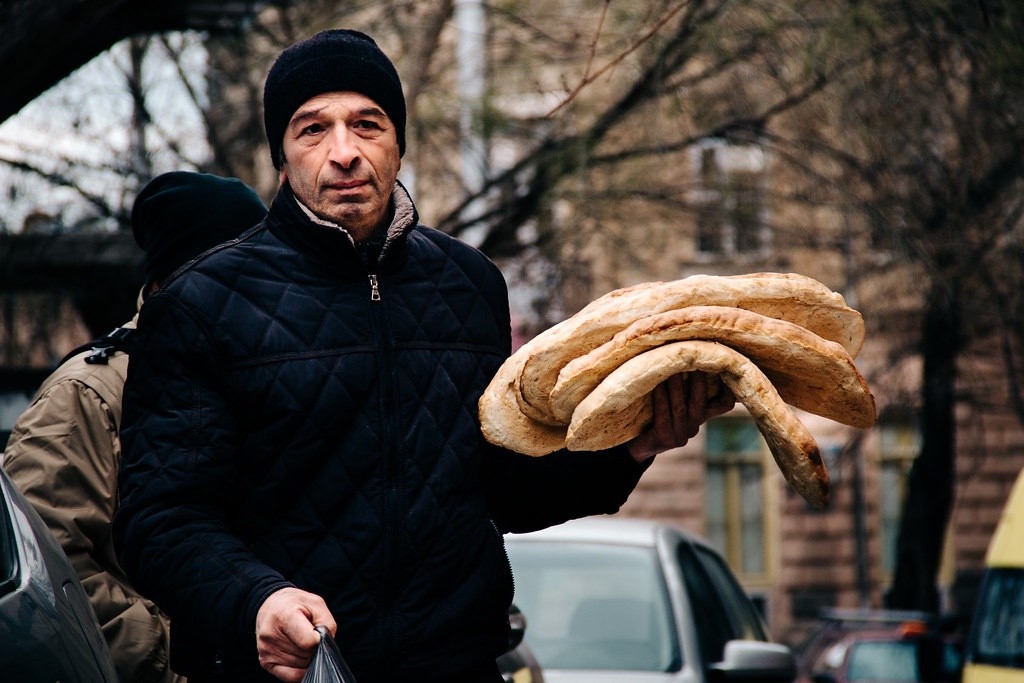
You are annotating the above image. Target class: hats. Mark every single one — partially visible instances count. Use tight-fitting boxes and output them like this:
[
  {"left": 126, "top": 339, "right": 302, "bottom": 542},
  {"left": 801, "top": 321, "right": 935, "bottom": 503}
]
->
[
  {"left": 263, "top": 29, "right": 406, "bottom": 173},
  {"left": 132, "top": 172, "right": 269, "bottom": 282}
]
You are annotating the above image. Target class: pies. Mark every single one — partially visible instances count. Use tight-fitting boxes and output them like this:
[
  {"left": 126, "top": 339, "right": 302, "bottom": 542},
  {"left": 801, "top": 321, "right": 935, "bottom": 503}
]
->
[{"left": 476, "top": 271, "right": 877, "bottom": 506}]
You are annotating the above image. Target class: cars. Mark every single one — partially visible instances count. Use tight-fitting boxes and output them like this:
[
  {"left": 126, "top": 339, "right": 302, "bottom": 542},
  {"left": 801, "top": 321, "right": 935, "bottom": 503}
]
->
[
  {"left": 798, "top": 619, "right": 959, "bottom": 683},
  {"left": 0, "top": 465, "right": 118, "bottom": 683},
  {"left": 502, "top": 518, "right": 797, "bottom": 683},
  {"left": 960, "top": 463, "right": 1024, "bottom": 683}
]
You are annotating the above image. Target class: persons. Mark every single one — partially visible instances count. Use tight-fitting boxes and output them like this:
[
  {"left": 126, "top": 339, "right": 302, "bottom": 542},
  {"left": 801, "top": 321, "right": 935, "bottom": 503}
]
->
[
  {"left": 3, "top": 171, "right": 271, "bottom": 683},
  {"left": 112, "top": 29, "right": 734, "bottom": 683}
]
